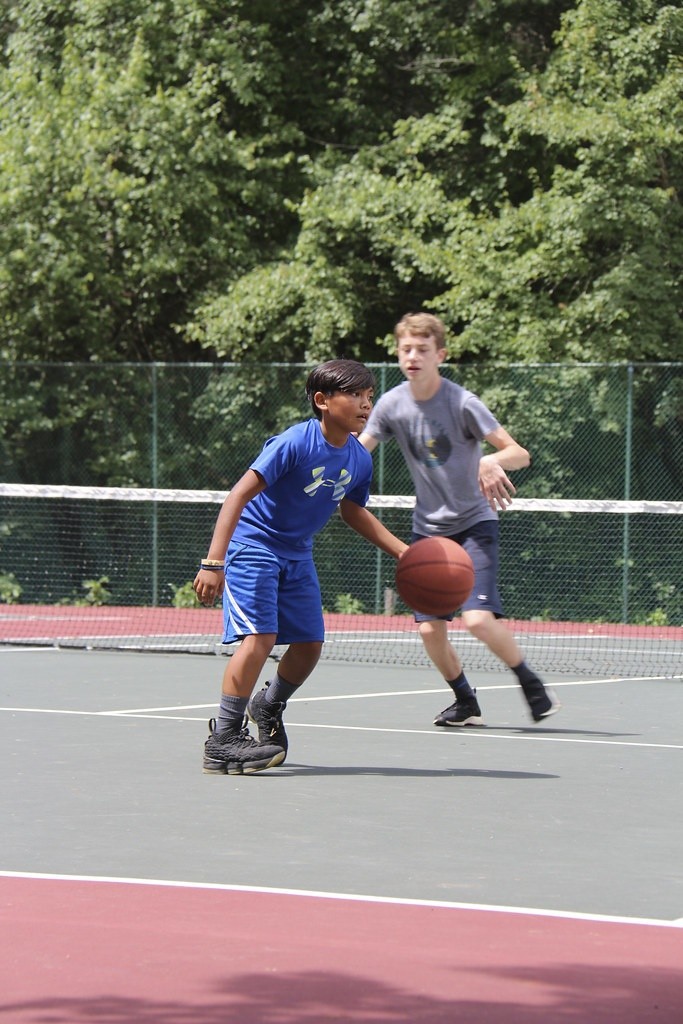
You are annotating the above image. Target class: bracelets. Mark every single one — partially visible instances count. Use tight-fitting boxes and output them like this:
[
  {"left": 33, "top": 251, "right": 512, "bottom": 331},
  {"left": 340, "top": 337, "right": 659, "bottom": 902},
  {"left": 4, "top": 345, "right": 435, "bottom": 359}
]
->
[
  {"left": 198, "top": 563, "right": 226, "bottom": 570},
  {"left": 201, "top": 556, "right": 227, "bottom": 567}
]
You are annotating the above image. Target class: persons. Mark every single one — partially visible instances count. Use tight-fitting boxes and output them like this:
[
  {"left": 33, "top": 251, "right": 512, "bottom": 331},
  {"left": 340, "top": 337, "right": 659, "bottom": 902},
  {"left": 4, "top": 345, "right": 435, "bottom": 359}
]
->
[
  {"left": 191, "top": 357, "right": 448, "bottom": 774},
  {"left": 346, "top": 309, "right": 562, "bottom": 727}
]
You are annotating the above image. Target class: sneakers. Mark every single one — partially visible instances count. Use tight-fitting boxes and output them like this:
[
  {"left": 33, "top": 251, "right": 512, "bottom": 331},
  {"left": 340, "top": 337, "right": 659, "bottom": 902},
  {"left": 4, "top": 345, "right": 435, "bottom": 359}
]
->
[
  {"left": 202, "top": 718, "right": 286, "bottom": 776},
  {"left": 245, "top": 689, "right": 288, "bottom": 766},
  {"left": 433, "top": 689, "right": 487, "bottom": 727},
  {"left": 519, "top": 672, "right": 560, "bottom": 722}
]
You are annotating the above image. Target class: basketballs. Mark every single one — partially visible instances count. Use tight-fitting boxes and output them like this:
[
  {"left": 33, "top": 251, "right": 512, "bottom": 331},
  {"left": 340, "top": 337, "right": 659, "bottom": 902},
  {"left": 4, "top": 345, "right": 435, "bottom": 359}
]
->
[{"left": 397, "top": 536, "right": 474, "bottom": 617}]
